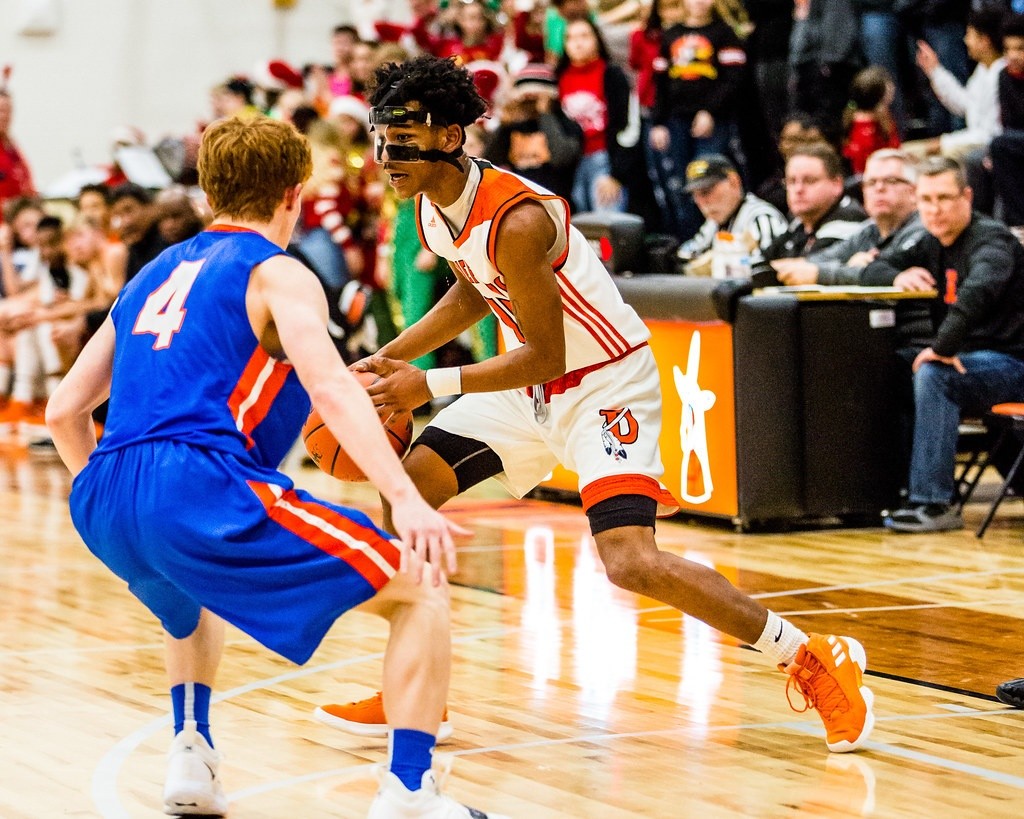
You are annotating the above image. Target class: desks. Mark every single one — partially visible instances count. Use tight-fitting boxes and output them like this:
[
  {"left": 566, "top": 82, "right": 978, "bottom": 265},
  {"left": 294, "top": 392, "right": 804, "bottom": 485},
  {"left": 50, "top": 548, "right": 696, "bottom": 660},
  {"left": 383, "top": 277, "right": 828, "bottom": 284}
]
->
[{"left": 496, "top": 275, "right": 940, "bottom": 534}]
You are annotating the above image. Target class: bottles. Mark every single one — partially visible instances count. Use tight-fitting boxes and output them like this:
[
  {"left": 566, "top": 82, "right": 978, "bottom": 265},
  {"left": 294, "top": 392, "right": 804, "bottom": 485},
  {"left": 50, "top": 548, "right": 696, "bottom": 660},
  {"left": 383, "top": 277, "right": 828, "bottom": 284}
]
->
[{"left": 710, "top": 230, "right": 752, "bottom": 281}]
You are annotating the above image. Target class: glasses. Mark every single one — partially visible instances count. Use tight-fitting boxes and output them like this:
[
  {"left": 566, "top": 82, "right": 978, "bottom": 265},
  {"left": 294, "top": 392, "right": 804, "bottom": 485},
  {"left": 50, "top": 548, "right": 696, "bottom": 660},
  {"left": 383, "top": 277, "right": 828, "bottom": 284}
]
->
[{"left": 861, "top": 176, "right": 911, "bottom": 188}]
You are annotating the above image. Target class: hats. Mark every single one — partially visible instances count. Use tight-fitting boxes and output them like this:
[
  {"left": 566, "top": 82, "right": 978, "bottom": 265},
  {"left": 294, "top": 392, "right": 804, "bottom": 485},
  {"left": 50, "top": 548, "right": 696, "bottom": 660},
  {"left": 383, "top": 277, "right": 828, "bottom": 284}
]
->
[
  {"left": 329, "top": 93, "right": 374, "bottom": 143},
  {"left": 684, "top": 155, "right": 733, "bottom": 192},
  {"left": 111, "top": 125, "right": 145, "bottom": 146},
  {"left": 510, "top": 65, "right": 559, "bottom": 101}
]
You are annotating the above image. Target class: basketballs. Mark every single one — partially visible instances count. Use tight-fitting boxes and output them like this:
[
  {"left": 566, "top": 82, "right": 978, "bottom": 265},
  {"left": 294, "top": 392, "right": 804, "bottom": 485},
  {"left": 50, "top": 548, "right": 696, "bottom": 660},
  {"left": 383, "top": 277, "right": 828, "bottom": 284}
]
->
[{"left": 300, "top": 367, "right": 413, "bottom": 481}]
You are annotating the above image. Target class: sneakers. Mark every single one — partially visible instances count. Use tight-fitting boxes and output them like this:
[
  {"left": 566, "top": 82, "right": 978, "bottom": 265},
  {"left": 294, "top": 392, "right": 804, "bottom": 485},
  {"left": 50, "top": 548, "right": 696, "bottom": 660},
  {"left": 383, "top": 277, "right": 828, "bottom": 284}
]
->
[
  {"left": 162, "top": 730, "right": 228, "bottom": 817},
  {"left": 776, "top": 632, "right": 874, "bottom": 753},
  {"left": 996, "top": 677, "right": 1024, "bottom": 709},
  {"left": 312, "top": 690, "right": 453, "bottom": 741},
  {"left": 884, "top": 500, "right": 963, "bottom": 533},
  {"left": 365, "top": 768, "right": 488, "bottom": 819}
]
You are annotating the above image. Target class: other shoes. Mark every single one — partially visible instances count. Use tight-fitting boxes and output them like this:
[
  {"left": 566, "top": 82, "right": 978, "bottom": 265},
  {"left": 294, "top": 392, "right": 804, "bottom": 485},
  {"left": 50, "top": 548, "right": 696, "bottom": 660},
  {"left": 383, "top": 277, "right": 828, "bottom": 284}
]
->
[
  {"left": 30, "top": 439, "right": 59, "bottom": 456},
  {"left": 412, "top": 401, "right": 431, "bottom": 417}
]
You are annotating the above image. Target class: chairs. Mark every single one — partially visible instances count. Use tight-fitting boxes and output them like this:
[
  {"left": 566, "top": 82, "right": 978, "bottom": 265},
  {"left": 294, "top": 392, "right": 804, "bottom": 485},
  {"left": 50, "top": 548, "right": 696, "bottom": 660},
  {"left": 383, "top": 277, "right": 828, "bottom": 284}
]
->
[{"left": 948, "top": 399, "right": 1024, "bottom": 538}]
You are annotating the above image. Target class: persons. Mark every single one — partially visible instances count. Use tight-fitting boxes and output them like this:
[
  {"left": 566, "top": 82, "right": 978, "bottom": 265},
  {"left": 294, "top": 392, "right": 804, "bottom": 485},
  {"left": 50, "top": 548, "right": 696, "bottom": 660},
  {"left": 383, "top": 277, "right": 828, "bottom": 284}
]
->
[
  {"left": 0, "top": 0, "right": 1024, "bottom": 533},
  {"left": 313, "top": 57, "right": 877, "bottom": 755},
  {"left": 44, "top": 114, "right": 515, "bottom": 819}
]
